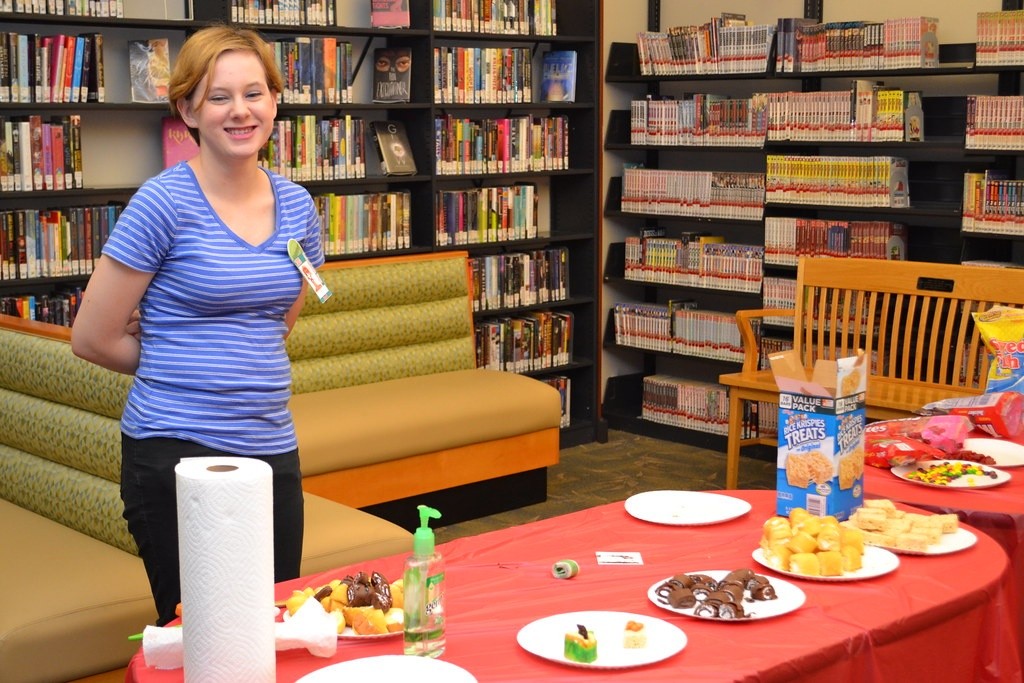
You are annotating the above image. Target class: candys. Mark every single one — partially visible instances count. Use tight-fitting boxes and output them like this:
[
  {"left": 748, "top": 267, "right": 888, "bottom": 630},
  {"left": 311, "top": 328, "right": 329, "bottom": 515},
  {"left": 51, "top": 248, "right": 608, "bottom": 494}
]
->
[{"left": 902, "top": 462, "right": 997, "bottom": 485}]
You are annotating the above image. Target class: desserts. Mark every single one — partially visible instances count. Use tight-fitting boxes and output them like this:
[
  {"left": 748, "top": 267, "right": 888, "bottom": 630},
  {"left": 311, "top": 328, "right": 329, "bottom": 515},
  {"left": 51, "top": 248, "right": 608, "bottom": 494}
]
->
[
  {"left": 565, "top": 624, "right": 597, "bottom": 663},
  {"left": 757, "top": 508, "right": 864, "bottom": 575},
  {"left": 655, "top": 569, "right": 777, "bottom": 619}
]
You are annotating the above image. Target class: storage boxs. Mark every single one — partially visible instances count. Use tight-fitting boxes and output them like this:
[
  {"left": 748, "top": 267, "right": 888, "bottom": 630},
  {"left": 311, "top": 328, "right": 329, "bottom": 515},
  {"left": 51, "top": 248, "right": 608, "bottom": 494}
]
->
[{"left": 768, "top": 349, "right": 866, "bottom": 522}]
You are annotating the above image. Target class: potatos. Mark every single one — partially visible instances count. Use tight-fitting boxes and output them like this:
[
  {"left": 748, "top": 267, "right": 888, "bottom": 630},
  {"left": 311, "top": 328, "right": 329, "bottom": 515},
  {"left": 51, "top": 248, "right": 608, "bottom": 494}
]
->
[{"left": 286, "top": 579, "right": 404, "bottom": 635}]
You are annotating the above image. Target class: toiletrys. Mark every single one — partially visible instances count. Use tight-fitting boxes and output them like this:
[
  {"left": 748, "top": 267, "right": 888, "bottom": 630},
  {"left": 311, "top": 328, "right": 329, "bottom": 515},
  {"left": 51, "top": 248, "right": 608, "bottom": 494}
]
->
[{"left": 402, "top": 504, "right": 449, "bottom": 660}]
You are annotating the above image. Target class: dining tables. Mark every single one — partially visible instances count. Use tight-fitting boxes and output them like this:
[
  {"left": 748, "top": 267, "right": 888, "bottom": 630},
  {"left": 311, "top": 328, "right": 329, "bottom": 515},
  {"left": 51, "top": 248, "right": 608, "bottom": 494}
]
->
[
  {"left": 128, "top": 490, "right": 1009, "bottom": 683},
  {"left": 865, "top": 432, "right": 1024, "bottom": 546}
]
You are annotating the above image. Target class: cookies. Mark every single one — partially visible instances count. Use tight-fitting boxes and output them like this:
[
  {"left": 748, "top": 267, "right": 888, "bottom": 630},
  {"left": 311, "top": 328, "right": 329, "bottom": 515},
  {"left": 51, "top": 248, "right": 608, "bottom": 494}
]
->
[
  {"left": 785, "top": 452, "right": 833, "bottom": 488},
  {"left": 841, "top": 500, "right": 958, "bottom": 551},
  {"left": 838, "top": 448, "right": 864, "bottom": 490}
]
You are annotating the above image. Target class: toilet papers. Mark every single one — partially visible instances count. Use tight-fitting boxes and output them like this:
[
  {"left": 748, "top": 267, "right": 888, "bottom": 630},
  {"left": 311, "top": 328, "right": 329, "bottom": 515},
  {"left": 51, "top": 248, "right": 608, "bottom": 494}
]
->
[{"left": 174, "top": 455, "right": 278, "bottom": 683}]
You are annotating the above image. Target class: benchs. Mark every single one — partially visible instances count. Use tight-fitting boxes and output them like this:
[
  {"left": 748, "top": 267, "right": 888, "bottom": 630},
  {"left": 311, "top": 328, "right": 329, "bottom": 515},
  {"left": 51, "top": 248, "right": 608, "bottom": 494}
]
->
[
  {"left": 0, "top": 314, "right": 136, "bottom": 683},
  {"left": 285, "top": 250, "right": 561, "bottom": 578},
  {"left": 720, "top": 257, "right": 1024, "bottom": 491}
]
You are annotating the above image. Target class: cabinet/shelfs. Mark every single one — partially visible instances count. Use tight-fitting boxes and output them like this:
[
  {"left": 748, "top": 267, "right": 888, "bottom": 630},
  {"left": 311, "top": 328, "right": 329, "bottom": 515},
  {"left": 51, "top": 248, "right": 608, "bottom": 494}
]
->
[
  {"left": 0, "top": 0, "right": 608, "bottom": 451},
  {"left": 600, "top": 43, "right": 1024, "bottom": 464}
]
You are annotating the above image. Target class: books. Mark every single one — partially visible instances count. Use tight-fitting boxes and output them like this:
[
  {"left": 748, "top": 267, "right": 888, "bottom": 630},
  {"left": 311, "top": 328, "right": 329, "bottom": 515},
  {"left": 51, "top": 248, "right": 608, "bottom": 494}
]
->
[
  {"left": 606, "top": 9, "right": 1024, "bottom": 448},
  {"left": 0, "top": 0, "right": 578, "bottom": 440}
]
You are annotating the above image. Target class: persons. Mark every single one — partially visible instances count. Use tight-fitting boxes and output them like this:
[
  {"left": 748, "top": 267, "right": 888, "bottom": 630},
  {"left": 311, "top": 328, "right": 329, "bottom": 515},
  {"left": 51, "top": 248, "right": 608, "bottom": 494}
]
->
[{"left": 72, "top": 30, "right": 326, "bottom": 623}]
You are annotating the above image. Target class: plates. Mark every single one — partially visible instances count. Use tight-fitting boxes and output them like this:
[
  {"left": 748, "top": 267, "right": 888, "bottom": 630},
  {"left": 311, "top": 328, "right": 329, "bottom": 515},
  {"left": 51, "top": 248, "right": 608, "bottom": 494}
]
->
[
  {"left": 516, "top": 611, "right": 688, "bottom": 669},
  {"left": 624, "top": 490, "right": 752, "bottom": 526},
  {"left": 647, "top": 570, "right": 807, "bottom": 621},
  {"left": 282, "top": 611, "right": 404, "bottom": 638},
  {"left": 960, "top": 438, "right": 1024, "bottom": 467},
  {"left": 751, "top": 546, "right": 901, "bottom": 581},
  {"left": 890, "top": 459, "right": 1011, "bottom": 489},
  {"left": 862, "top": 528, "right": 977, "bottom": 555},
  {"left": 294, "top": 655, "right": 479, "bottom": 683}
]
